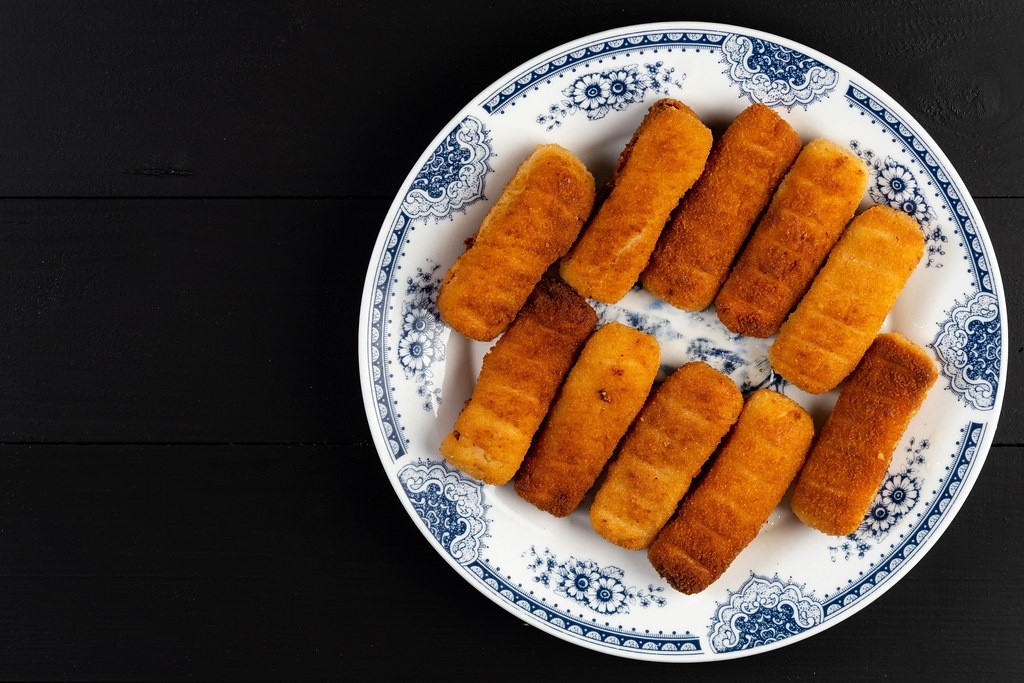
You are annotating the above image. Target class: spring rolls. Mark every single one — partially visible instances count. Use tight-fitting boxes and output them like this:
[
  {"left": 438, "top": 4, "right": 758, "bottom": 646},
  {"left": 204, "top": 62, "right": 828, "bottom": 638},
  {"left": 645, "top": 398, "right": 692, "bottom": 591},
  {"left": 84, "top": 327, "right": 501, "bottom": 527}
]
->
[{"left": 437, "top": 99, "right": 939, "bottom": 593}]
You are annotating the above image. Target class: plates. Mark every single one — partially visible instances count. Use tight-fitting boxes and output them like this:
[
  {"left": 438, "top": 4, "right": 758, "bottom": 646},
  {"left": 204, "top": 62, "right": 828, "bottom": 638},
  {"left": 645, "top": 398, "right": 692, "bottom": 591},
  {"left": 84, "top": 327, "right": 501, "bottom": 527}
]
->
[{"left": 356, "top": 18, "right": 1009, "bottom": 663}]
